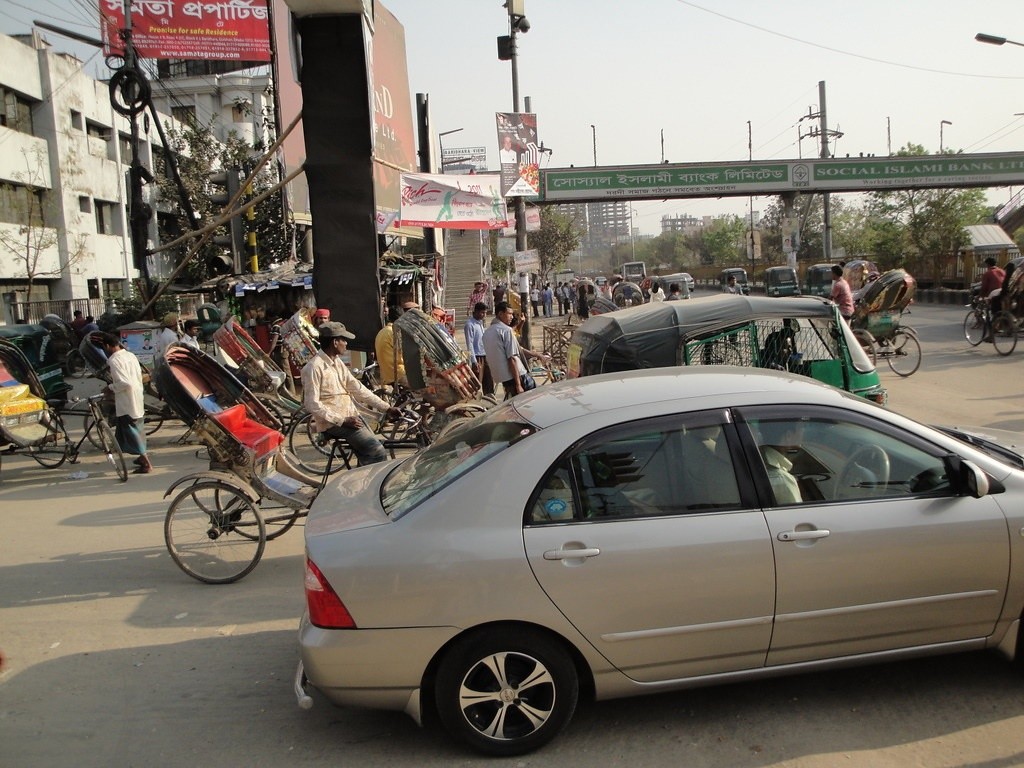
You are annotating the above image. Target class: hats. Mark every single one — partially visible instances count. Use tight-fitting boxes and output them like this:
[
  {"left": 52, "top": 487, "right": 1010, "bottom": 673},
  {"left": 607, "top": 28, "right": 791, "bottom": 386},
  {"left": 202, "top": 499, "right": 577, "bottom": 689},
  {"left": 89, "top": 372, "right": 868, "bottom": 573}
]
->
[
  {"left": 403, "top": 301, "right": 420, "bottom": 308},
  {"left": 318, "top": 321, "right": 356, "bottom": 340},
  {"left": 315, "top": 309, "right": 331, "bottom": 318}
]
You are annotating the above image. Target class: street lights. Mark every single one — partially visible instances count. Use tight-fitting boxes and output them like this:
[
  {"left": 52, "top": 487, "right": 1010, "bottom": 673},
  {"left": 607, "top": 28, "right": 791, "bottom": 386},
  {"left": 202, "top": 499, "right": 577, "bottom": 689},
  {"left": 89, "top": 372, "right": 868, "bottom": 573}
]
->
[
  {"left": 660, "top": 128, "right": 666, "bottom": 164},
  {"left": 495, "top": 0, "right": 532, "bottom": 375},
  {"left": 939, "top": 120, "right": 959, "bottom": 160},
  {"left": 747, "top": 120, "right": 753, "bottom": 162},
  {"left": 591, "top": 124, "right": 600, "bottom": 167}
]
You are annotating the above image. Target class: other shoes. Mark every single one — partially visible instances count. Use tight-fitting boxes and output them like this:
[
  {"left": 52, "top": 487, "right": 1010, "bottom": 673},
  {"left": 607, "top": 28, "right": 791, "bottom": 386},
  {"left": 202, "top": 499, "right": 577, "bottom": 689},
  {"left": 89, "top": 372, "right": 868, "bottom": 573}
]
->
[
  {"left": 970, "top": 318, "right": 984, "bottom": 330},
  {"left": 983, "top": 336, "right": 993, "bottom": 343}
]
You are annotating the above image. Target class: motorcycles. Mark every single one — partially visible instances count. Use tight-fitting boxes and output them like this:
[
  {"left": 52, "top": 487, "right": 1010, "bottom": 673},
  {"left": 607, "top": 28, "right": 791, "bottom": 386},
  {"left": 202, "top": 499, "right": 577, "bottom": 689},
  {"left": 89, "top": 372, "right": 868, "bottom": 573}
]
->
[
  {"left": 806, "top": 264, "right": 845, "bottom": 297},
  {"left": 763, "top": 266, "right": 803, "bottom": 297},
  {"left": 563, "top": 293, "right": 890, "bottom": 413},
  {"left": 721, "top": 268, "right": 750, "bottom": 296},
  {"left": 0, "top": 325, "right": 75, "bottom": 408}
]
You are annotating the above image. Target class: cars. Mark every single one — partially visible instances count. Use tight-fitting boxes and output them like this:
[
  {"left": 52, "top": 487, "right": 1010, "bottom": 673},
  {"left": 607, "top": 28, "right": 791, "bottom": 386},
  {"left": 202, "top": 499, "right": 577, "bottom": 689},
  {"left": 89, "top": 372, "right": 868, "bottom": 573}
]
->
[{"left": 294, "top": 362, "right": 1016, "bottom": 761}]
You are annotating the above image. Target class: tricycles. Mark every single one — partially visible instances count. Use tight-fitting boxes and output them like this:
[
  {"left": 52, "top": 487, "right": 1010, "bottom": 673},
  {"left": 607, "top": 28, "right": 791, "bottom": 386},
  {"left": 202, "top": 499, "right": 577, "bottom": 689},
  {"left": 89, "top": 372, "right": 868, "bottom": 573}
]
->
[
  {"left": 826, "top": 269, "right": 943, "bottom": 381},
  {"left": 153, "top": 332, "right": 432, "bottom": 584},
  {"left": 41, "top": 314, "right": 88, "bottom": 379},
  {"left": 0, "top": 340, "right": 130, "bottom": 481},
  {"left": 208, "top": 306, "right": 567, "bottom": 479}
]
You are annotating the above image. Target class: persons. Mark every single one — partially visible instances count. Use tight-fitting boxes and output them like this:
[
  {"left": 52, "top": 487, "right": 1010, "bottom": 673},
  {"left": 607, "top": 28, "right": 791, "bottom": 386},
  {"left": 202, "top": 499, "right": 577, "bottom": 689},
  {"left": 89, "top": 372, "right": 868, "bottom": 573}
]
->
[
  {"left": 663, "top": 283, "right": 681, "bottom": 301},
  {"left": 159, "top": 313, "right": 200, "bottom": 355},
  {"left": 530, "top": 281, "right": 571, "bottom": 318},
  {"left": 375, "top": 306, "right": 408, "bottom": 386},
  {"left": 464, "top": 302, "right": 494, "bottom": 395},
  {"left": 265, "top": 306, "right": 296, "bottom": 394},
  {"left": 316, "top": 309, "right": 330, "bottom": 324},
  {"left": 827, "top": 266, "right": 854, "bottom": 326},
  {"left": 466, "top": 281, "right": 488, "bottom": 317},
  {"left": 73, "top": 311, "right": 99, "bottom": 333},
  {"left": 122, "top": 340, "right": 129, "bottom": 349},
  {"left": 492, "top": 286, "right": 504, "bottom": 303},
  {"left": 99, "top": 333, "right": 154, "bottom": 473},
  {"left": 500, "top": 136, "right": 517, "bottom": 163},
  {"left": 759, "top": 418, "right": 804, "bottom": 505},
  {"left": 483, "top": 301, "right": 547, "bottom": 397},
  {"left": 142, "top": 338, "right": 152, "bottom": 350},
  {"left": 610, "top": 268, "right": 622, "bottom": 287},
  {"left": 601, "top": 281, "right": 612, "bottom": 302},
  {"left": 648, "top": 281, "right": 666, "bottom": 303},
  {"left": 980, "top": 257, "right": 1007, "bottom": 343},
  {"left": 300, "top": 322, "right": 403, "bottom": 467},
  {"left": 640, "top": 274, "right": 651, "bottom": 297},
  {"left": 723, "top": 275, "right": 742, "bottom": 343}
]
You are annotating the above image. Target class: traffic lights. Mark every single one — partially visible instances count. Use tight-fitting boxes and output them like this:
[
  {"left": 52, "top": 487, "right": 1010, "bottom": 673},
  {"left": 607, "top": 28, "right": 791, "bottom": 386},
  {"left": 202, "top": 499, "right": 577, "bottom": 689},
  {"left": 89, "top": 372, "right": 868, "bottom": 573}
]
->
[
  {"left": 207, "top": 173, "right": 238, "bottom": 248},
  {"left": 210, "top": 257, "right": 233, "bottom": 274}
]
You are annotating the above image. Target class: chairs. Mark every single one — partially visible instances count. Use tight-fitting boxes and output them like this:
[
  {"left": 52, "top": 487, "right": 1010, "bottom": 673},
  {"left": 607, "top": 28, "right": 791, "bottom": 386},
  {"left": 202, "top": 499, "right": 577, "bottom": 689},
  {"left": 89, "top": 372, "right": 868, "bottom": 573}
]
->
[
  {"left": 215, "top": 404, "right": 287, "bottom": 459},
  {"left": 253, "top": 359, "right": 290, "bottom": 391},
  {"left": 0, "top": 383, "right": 47, "bottom": 416},
  {"left": 682, "top": 414, "right": 721, "bottom": 505},
  {"left": 705, "top": 422, "right": 762, "bottom": 507}
]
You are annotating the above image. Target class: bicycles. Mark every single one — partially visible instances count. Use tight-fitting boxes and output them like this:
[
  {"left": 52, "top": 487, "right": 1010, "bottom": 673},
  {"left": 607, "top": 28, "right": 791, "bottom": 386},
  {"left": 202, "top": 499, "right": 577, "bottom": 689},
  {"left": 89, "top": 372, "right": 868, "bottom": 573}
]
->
[{"left": 962, "top": 293, "right": 1024, "bottom": 359}]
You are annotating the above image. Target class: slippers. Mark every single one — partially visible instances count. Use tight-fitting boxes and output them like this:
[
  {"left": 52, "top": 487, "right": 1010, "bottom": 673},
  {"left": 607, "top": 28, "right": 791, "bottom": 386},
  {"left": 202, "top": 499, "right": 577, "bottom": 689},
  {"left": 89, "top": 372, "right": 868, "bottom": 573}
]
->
[
  {"left": 132, "top": 465, "right": 152, "bottom": 473},
  {"left": 133, "top": 457, "right": 144, "bottom": 466}
]
